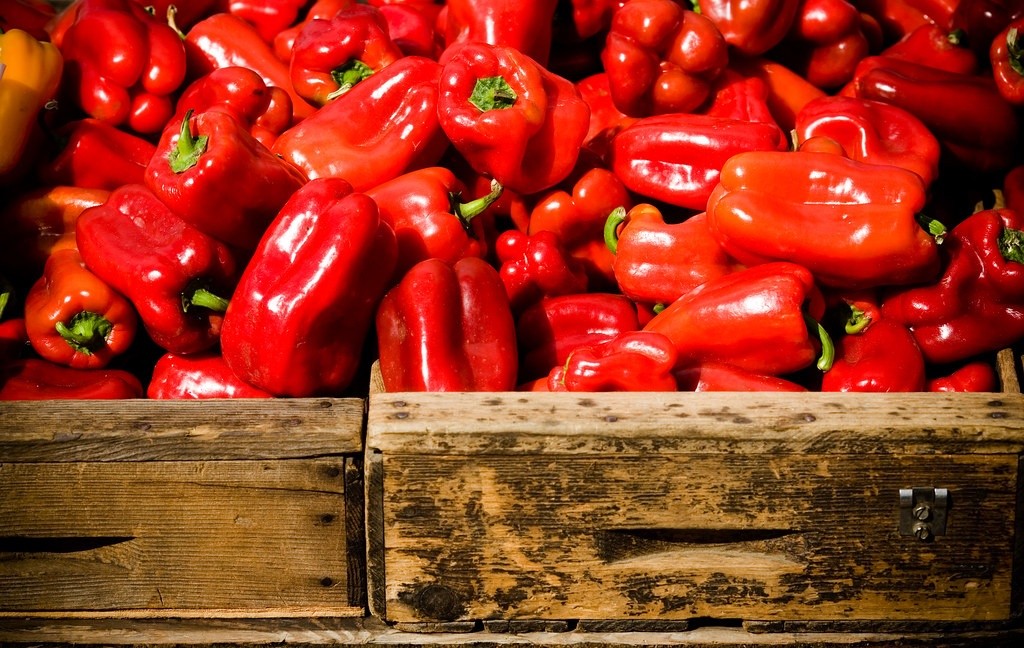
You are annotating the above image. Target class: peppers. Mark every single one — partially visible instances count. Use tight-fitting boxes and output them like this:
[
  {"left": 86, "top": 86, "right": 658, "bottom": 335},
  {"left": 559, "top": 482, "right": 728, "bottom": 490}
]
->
[{"left": 0, "top": 0, "right": 1024, "bottom": 403}]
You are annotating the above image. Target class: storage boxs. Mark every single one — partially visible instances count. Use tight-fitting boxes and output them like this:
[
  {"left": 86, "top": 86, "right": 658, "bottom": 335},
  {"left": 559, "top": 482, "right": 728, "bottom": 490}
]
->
[{"left": 0, "top": 310, "right": 1024, "bottom": 633}]
